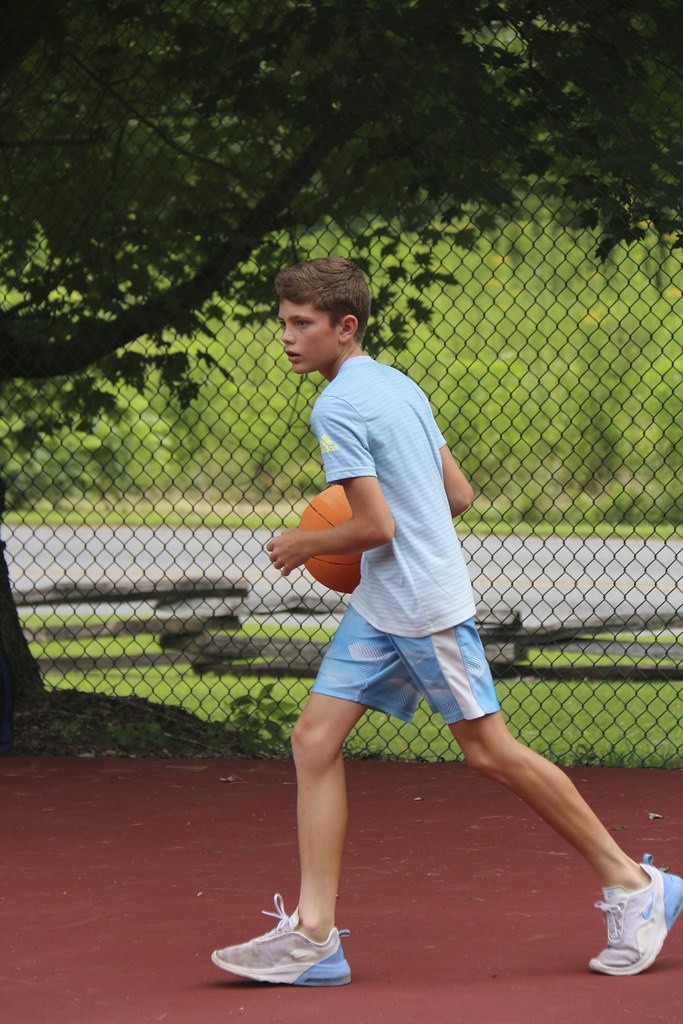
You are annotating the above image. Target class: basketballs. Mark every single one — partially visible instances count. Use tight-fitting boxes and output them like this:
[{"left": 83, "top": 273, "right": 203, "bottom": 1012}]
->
[{"left": 298, "top": 486, "right": 367, "bottom": 594}]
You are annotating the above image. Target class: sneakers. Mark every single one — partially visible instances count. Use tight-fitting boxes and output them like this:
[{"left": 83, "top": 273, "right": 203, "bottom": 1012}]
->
[
  {"left": 586, "top": 854, "right": 683, "bottom": 979},
  {"left": 211, "top": 908, "right": 352, "bottom": 986}
]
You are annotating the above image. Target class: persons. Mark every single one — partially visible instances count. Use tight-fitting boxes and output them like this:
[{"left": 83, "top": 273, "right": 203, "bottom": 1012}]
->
[{"left": 208, "top": 259, "right": 683, "bottom": 991}]
